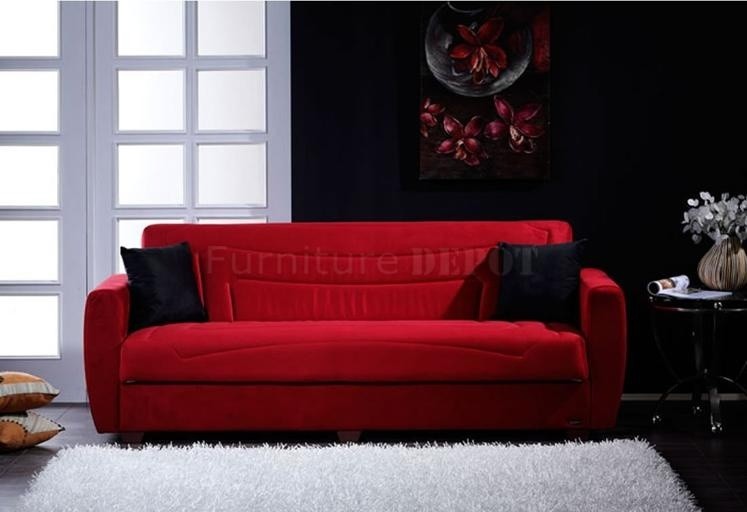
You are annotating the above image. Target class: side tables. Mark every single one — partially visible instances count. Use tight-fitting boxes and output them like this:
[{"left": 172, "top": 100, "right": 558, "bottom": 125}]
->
[{"left": 650, "top": 292, "right": 747, "bottom": 437}]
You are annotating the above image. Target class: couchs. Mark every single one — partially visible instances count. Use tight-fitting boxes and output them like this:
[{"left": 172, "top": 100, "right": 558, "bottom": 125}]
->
[{"left": 85, "top": 219, "right": 627, "bottom": 443}]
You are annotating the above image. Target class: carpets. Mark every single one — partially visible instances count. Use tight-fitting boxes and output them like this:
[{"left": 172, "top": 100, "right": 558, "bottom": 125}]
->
[{"left": 17, "top": 437, "right": 699, "bottom": 512}]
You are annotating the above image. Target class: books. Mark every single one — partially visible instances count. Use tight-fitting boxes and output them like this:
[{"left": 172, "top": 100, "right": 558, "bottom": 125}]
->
[{"left": 646, "top": 273, "right": 734, "bottom": 303}]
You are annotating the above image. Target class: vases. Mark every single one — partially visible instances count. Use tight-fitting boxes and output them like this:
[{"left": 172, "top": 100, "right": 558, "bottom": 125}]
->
[{"left": 698, "top": 235, "right": 747, "bottom": 291}]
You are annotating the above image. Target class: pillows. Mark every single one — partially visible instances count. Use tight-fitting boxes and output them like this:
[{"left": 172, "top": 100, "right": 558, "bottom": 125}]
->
[
  {"left": 491, "top": 238, "right": 589, "bottom": 330},
  {"left": 0, "top": 371, "right": 61, "bottom": 411},
  {"left": 121, "top": 240, "right": 209, "bottom": 336},
  {"left": 0, "top": 411, "right": 65, "bottom": 450}
]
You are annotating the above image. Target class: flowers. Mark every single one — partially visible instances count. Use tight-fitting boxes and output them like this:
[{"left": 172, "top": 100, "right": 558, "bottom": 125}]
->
[{"left": 682, "top": 190, "right": 747, "bottom": 245}]
